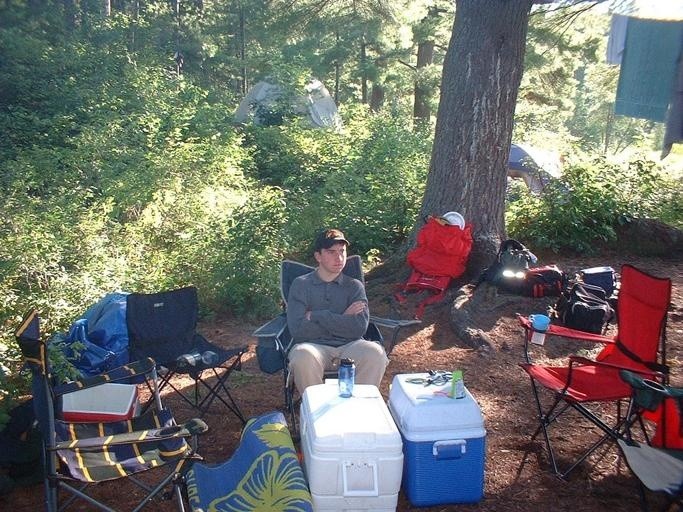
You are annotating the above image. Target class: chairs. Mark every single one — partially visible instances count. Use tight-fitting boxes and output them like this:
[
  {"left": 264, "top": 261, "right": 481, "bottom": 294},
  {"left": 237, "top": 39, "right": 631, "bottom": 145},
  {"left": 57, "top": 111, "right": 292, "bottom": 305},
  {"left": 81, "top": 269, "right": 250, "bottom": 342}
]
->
[
  {"left": 126, "top": 287, "right": 249, "bottom": 425},
  {"left": 252, "top": 256, "right": 422, "bottom": 432},
  {"left": 516, "top": 265, "right": 671, "bottom": 503},
  {"left": 12, "top": 308, "right": 209, "bottom": 512}
]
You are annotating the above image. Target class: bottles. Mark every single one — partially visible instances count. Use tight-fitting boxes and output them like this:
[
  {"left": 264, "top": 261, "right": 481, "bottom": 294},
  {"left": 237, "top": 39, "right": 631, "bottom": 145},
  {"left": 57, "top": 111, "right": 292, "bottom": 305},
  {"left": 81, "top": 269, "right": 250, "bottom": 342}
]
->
[
  {"left": 338, "top": 356, "right": 356, "bottom": 398},
  {"left": 176, "top": 351, "right": 201, "bottom": 368},
  {"left": 451, "top": 369, "right": 466, "bottom": 399}
]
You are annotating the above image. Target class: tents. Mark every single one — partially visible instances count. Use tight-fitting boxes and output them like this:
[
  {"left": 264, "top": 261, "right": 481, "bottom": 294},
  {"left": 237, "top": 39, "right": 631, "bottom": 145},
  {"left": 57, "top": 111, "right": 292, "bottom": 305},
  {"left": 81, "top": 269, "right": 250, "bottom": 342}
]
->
[
  {"left": 234, "top": 72, "right": 344, "bottom": 134},
  {"left": 507, "top": 140, "right": 567, "bottom": 196}
]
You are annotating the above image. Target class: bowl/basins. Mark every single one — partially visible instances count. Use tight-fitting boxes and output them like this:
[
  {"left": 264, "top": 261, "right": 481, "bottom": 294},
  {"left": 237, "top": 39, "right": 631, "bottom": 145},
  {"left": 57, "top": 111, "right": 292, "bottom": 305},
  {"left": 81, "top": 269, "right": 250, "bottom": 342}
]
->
[{"left": 442, "top": 212, "right": 465, "bottom": 230}]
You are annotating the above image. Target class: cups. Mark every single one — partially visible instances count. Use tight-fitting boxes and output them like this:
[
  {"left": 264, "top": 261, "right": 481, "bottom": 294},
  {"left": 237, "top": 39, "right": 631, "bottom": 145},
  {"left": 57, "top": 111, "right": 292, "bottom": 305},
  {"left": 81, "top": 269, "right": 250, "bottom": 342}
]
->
[
  {"left": 530, "top": 314, "right": 551, "bottom": 331},
  {"left": 636, "top": 378, "right": 667, "bottom": 412},
  {"left": 203, "top": 351, "right": 218, "bottom": 365}
]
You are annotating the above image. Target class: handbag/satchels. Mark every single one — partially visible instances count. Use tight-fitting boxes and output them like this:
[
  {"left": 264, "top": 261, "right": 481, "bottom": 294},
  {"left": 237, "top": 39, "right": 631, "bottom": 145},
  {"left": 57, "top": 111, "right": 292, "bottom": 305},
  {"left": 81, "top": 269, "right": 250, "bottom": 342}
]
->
[
  {"left": 525, "top": 264, "right": 566, "bottom": 298},
  {"left": 480, "top": 239, "right": 537, "bottom": 291},
  {"left": 556, "top": 282, "right": 615, "bottom": 335}
]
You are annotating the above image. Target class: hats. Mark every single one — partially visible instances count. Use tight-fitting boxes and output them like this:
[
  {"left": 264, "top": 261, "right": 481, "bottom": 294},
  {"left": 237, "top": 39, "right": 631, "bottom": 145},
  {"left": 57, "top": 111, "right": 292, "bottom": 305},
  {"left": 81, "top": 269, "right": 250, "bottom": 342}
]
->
[{"left": 316, "top": 229, "right": 351, "bottom": 249}]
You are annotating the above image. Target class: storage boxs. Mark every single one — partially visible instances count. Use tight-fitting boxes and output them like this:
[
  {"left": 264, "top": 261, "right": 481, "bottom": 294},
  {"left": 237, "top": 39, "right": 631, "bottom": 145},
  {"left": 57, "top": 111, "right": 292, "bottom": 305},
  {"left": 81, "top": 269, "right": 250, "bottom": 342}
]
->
[
  {"left": 389, "top": 371, "right": 487, "bottom": 507},
  {"left": 62, "top": 383, "right": 142, "bottom": 423},
  {"left": 299, "top": 385, "right": 404, "bottom": 511}
]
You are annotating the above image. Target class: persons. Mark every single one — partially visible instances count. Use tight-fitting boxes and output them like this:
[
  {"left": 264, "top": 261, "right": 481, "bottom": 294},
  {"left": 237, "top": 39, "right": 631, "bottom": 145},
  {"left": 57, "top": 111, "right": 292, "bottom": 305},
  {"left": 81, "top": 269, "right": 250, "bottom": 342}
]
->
[{"left": 287, "top": 229, "right": 388, "bottom": 397}]
